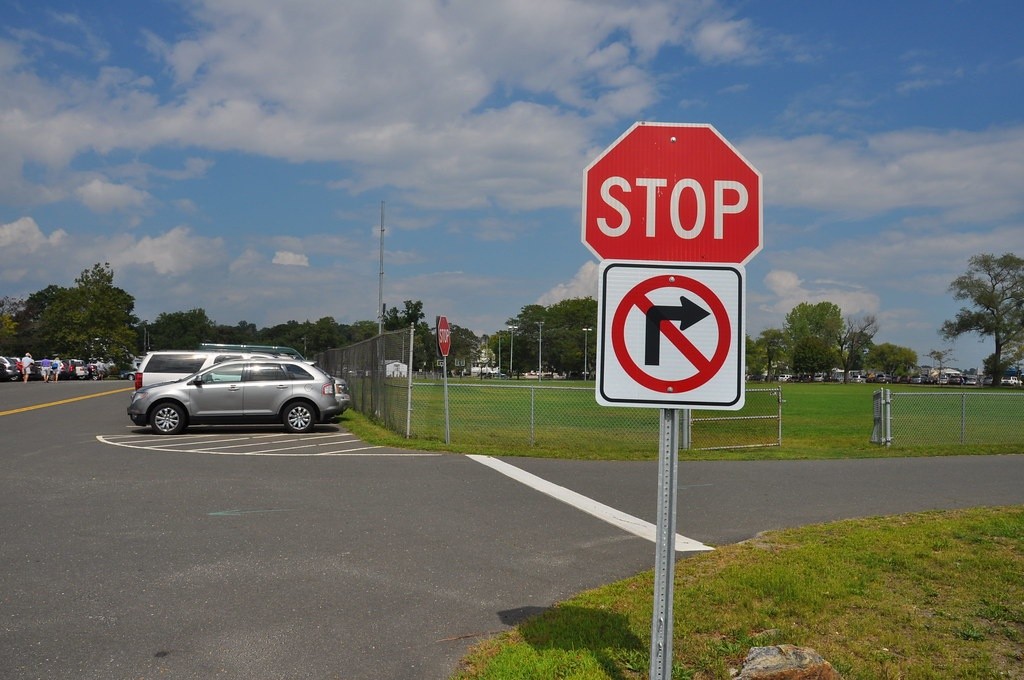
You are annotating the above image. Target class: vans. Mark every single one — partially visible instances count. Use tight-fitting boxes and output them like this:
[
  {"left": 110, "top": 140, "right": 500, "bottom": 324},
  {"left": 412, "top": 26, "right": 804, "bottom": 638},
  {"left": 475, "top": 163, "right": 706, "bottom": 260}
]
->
[{"left": 200, "top": 343, "right": 341, "bottom": 412}]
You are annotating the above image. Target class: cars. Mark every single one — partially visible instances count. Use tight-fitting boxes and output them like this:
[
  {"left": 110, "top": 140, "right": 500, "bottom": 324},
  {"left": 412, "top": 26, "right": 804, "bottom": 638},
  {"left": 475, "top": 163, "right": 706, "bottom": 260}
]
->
[
  {"left": 0, "top": 355, "right": 148, "bottom": 381},
  {"left": 439, "top": 368, "right": 597, "bottom": 381},
  {"left": 743, "top": 368, "right": 1021, "bottom": 385}
]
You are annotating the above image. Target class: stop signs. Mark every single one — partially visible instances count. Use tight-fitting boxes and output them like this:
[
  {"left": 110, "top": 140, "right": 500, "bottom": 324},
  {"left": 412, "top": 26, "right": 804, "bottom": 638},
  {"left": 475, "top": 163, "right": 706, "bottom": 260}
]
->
[
  {"left": 438, "top": 315, "right": 451, "bottom": 357},
  {"left": 579, "top": 120, "right": 765, "bottom": 265}
]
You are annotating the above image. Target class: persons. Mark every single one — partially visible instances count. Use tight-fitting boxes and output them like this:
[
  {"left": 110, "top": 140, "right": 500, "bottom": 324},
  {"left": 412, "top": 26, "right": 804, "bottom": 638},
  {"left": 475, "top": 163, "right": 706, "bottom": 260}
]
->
[{"left": 21, "top": 352, "right": 107, "bottom": 383}]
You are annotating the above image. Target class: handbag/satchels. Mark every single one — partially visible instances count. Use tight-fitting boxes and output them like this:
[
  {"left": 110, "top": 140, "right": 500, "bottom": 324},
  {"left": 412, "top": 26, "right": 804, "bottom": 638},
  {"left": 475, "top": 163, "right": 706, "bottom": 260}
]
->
[
  {"left": 21, "top": 367, "right": 26, "bottom": 375},
  {"left": 51, "top": 363, "right": 57, "bottom": 369}
]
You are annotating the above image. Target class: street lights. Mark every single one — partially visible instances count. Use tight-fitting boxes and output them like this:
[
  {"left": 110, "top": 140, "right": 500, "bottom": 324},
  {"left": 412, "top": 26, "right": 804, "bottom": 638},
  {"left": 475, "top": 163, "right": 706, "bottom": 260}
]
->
[
  {"left": 582, "top": 328, "right": 592, "bottom": 380},
  {"left": 508, "top": 325, "right": 518, "bottom": 379},
  {"left": 534, "top": 321, "right": 545, "bottom": 380}
]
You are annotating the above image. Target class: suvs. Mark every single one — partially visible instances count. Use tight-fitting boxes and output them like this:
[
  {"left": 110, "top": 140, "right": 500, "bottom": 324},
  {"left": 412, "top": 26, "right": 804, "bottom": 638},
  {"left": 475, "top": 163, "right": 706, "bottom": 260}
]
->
[
  {"left": 137, "top": 350, "right": 303, "bottom": 417},
  {"left": 200, "top": 353, "right": 351, "bottom": 429},
  {"left": 126, "top": 358, "right": 340, "bottom": 433}
]
